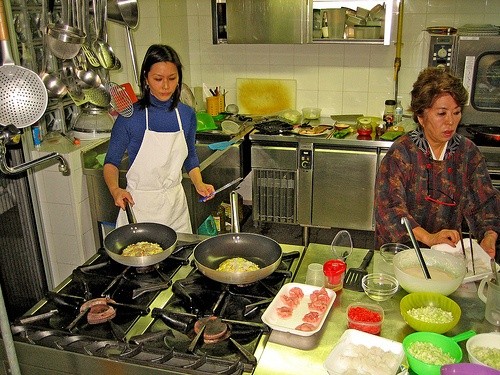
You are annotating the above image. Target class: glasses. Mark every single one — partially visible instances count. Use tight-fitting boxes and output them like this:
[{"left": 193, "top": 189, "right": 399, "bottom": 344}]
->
[{"left": 425, "top": 167, "right": 457, "bottom": 208}]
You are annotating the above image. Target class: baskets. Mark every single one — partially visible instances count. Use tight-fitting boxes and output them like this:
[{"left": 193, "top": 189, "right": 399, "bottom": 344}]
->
[{"left": 206, "top": 96, "right": 224, "bottom": 116}]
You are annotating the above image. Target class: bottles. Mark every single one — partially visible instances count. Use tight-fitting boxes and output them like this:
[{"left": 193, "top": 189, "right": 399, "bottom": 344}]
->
[{"left": 356, "top": 95, "right": 403, "bottom": 136}]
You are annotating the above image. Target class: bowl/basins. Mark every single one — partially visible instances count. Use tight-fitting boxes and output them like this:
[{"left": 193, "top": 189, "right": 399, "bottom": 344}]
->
[
  {"left": 380, "top": 243, "right": 410, "bottom": 264},
  {"left": 392, "top": 248, "right": 466, "bottom": 296},
  {"left": 324, "top": 329, "right": 405, "bottom": 375},
  {"left": 439, "top": 363, "right": 499, "bottom": 375},
  {"left": 221, "top": 121, "right": 240, "bottom": 134},
  {"left": 399, "top": 291, "right": 462, "bottom": 332},
  {"left": 302, "top": 107, "right": 322, "bottom": 120},
  {"left": 464, "top": 331, "right": 500, "bottom": 370},
  {"left": 346, "top": 302, "right": 384, "bottom": 335},
  {"left": 195, "top": 112, "right": 218, "bottom": 132},
  {"left": 313, "top": 2, "right": 386, "bottom": 41},
  {"left": 363, "top": 273, "right": 398, "bottom": 302}
]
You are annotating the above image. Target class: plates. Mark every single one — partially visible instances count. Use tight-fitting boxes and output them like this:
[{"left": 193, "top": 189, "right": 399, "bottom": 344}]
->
[
  {"left": 262, "top": 282, "right": 336, "bottom": 336},
  {"left": 293, "top": 127, "right": 329, "bottom": 136},
  {"left": 431, "top": 237, "right": 500, "bottom": 281}
]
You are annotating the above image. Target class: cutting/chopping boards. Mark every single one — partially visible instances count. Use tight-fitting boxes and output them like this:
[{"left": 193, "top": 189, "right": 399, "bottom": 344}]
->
[{"left": 237, "top": 78, "right": 297, "bottom": 114}]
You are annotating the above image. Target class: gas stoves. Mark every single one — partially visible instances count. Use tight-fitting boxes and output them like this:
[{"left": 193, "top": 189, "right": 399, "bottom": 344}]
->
[{"left": 7, "top": 236, "right": 302, "bottom": 375}]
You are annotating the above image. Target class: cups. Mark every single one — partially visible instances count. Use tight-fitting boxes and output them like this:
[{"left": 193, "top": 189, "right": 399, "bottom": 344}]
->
[{"left": 305, "top": 263, "right": 325, "bottom": 288}]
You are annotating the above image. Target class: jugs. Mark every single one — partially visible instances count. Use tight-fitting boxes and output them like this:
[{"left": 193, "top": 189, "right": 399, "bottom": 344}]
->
[
  {"left": 319, "top": 8, "right": 347, "bottom": 38},
  {"left": 323, "top": 260, "right": 347, "bottom": 297},
  {"left": 478, "top": 272, "right": 500, "bottom": 327}
]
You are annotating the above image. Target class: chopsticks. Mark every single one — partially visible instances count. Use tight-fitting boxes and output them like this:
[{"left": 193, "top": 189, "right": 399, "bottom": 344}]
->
[
  {"left": 459, "top": 226, "right": 476, "bottom": 275},
  {"left": 326, "top": 128, "right": 335, "bottom": 140}
]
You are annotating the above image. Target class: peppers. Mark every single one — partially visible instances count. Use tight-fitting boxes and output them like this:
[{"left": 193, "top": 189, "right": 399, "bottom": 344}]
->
[{"left": 347, "top": 306, "right": 381, "bottom": 334}]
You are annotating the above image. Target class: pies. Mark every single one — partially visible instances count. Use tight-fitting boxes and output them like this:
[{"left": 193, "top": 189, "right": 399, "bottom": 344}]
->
[
  {"left": 121, "top": 241, "right": 163, "bottom": 256},
  {"left": 212, "top": 256, "right": 259, "bottom": 272}
]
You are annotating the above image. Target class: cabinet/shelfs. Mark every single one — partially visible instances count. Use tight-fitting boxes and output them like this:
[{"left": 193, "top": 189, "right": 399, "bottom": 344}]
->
[{"left": 213, "top": 0, "right": 399, "bottom": 46}]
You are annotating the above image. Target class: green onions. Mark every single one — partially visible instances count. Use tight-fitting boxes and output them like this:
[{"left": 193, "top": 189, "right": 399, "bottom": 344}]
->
[
  {"left": 332, "top": 126, "right": 354, "bottom": 139},
  {"left": 469, "top": 346, "right": 500, "bottom": 372}
]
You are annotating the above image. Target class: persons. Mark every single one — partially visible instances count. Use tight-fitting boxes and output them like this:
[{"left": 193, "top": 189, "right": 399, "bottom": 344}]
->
[
  {"left": 374, "top": 68, "right": 500, "bottom": 259},
  {"left": 103, "top": 43, "right": 214, "bottom": 234}
]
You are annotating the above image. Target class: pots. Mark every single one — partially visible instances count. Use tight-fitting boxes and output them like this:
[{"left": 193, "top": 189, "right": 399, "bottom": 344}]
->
[
  {"left": 102, "top": 199, "right": 178, "bottom": 265},
  {"left": 193, "top": 191, "right": 283, "bottom": 282}
]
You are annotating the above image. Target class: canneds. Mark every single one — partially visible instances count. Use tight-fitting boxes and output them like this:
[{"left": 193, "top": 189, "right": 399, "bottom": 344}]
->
[
  {"left": 357, "top": 118, "right": 372, "bottom": 136},
  {"left": 382, "top": 100, "right": 397, "bottom": 128},
  {"left": 32, "top": 126, "right": 40, "bottom": 145}
]
